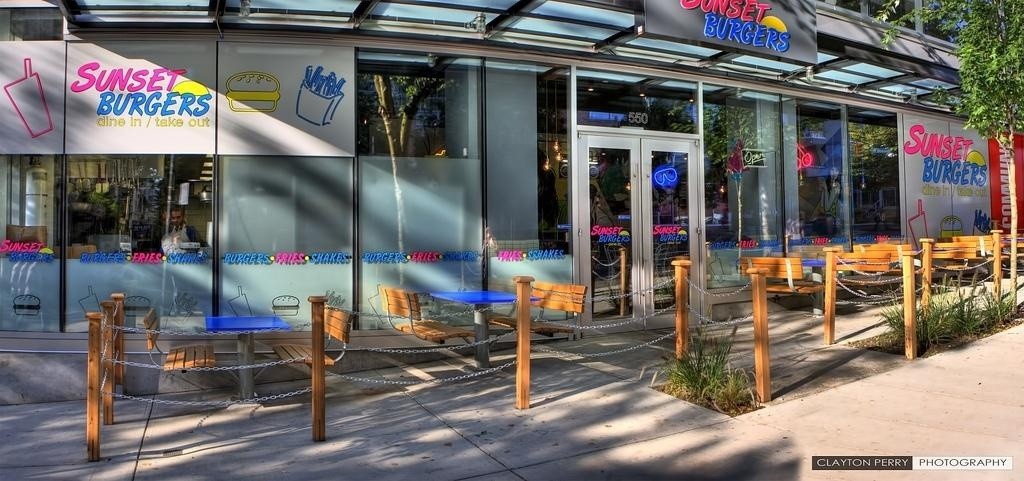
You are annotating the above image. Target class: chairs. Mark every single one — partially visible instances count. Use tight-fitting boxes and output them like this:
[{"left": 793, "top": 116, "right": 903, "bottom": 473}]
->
[{"left": 378, "top": 281, "right": 588, "bottom": 376}]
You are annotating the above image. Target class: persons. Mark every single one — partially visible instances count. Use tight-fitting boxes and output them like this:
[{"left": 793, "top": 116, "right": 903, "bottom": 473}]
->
[
  {"left": 160, "top": 204, "right": 204, "bottom": 254},
  {"left": 809, "top": 166, "right": 840, "bottom": 221}
]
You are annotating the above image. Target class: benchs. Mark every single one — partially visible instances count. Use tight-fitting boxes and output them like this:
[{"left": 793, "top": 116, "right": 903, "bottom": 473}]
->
[
  {"left": 739, "top": 235, "right": 1023, "bottom": 316},
  {"left": 143, "top": 308, "right": 352, "bottom": 400}
]
